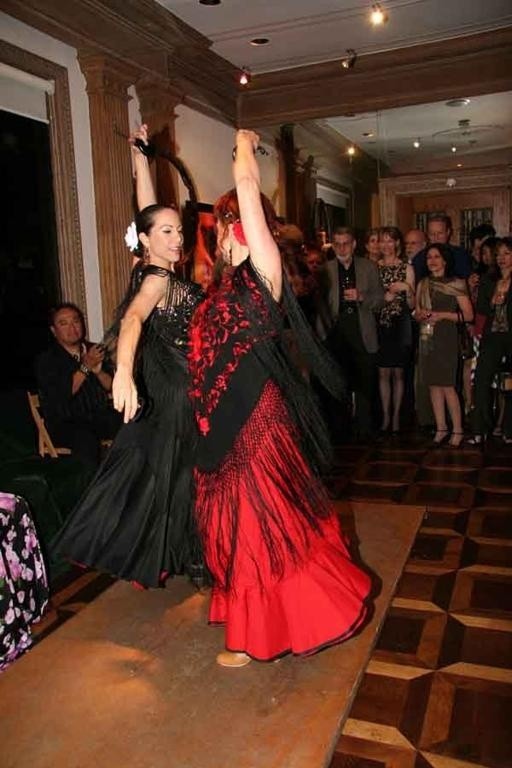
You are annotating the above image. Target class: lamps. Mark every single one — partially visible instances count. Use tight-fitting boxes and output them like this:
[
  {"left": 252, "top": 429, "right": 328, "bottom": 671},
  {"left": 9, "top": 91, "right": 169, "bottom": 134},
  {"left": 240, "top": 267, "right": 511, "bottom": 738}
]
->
[
  {"left": 238, "top": 65, "right": 253, "bottom": 84},
  {"left": 341, "top": 47, "right": 359, "bottom": 71}
]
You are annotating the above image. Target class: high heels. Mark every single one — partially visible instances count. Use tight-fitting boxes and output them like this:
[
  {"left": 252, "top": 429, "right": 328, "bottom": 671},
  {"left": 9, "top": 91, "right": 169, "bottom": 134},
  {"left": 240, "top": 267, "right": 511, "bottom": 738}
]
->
[
  {"left": 427, "top": 429, "right": 489, "bottom": 449},
  {"left": 378, "top": 427, "right": 400, "bottom": 438}
]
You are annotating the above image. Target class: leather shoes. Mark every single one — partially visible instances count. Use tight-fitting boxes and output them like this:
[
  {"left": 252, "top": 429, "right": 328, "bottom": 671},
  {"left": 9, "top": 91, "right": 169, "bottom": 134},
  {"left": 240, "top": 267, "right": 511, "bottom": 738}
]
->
[{"left": 215, "top": 652, "right": 252, "bottom": 667}]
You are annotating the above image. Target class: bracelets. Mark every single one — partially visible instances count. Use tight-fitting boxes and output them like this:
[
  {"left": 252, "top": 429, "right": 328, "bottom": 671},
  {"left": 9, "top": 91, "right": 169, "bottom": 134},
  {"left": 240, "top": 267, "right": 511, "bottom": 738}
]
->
[{"left": 80, "top": 365, "right": 91, "bottom": 377}]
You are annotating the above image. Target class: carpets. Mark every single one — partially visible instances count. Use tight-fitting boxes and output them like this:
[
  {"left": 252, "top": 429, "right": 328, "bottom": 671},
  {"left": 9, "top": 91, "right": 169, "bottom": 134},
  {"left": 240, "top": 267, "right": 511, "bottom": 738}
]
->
[{"left": 0, "top": 500, "right": 425, "bottom": 766}]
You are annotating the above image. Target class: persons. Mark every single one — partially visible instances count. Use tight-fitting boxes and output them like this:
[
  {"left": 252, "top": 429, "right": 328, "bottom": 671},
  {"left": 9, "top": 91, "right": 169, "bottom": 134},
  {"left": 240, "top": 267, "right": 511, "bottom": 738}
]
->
[
  {"left": 47, "top": 117, "right": 210, "bottom": 602},
  {"left": 189, "top": 124, "right": 373, "bottom": 670},
  {"left": 27, "top": 301, "right": 117, "bottom": 479},
  {"left": 271, "top": 205, "right": 511, "bottom": 448}
]
